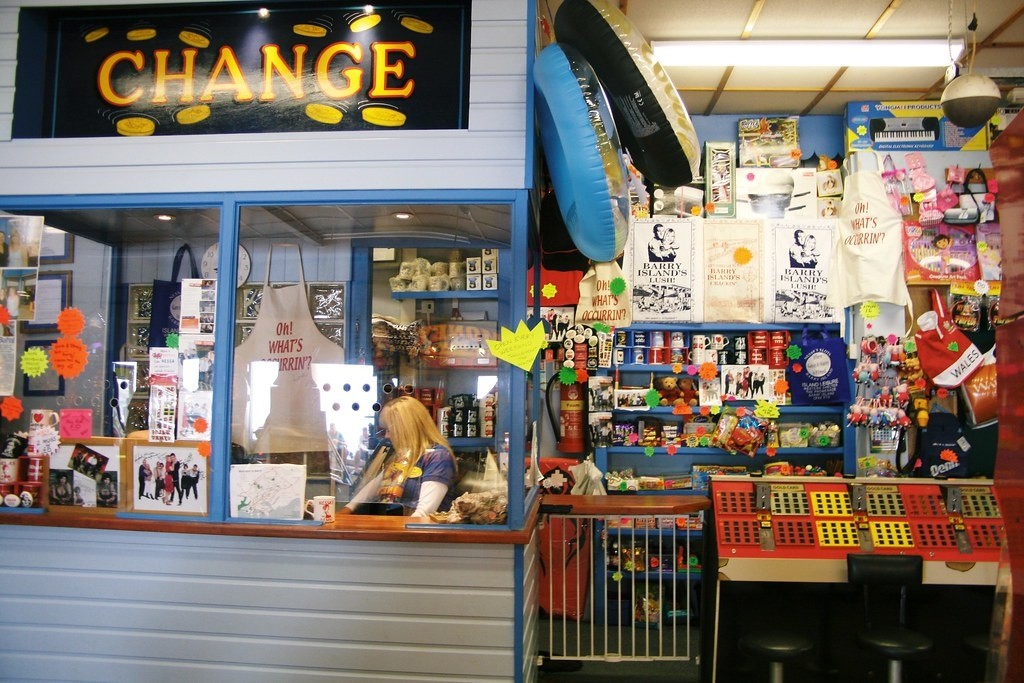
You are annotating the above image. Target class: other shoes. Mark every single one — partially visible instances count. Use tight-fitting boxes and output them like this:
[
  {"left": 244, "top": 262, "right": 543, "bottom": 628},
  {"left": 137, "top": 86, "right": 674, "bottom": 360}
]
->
[
  {"left": 186, "top": 496, "right": 188, "bottom": 499},
  {"left": 141, "top": 494, "right": 145, "bottom": 496},
  {"left": 145, "top": 493, "right": 150, "bottom": 499},
  {"left": 176, "top": 504, "right": 182, "bottom": 506},
  {"left": 350, "top": 470, "right": 359, "bottom": 476},
  {"left": 159, "top": 496, "right": 162, "bottom": 497},
  {"left": 162, "top": 498, "right": 165, "bottom": 503},
  {"left": 139, "top": 497, "right": 141, "bottom": 499},
  {"left": 155, "top": 498, "right": 159, "bottom": 500},
  {"left": 150, "top": 494, "right": 154, "bottom": 500},
  {"left": 166, "top": 503, "right": 171, "bottom": 505},
  {"left": 169, "top": 500, "right": 172, "bottom": 502}
]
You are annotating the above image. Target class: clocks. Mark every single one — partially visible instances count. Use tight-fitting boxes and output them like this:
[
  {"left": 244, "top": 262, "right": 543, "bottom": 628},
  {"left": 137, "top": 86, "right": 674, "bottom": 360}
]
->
[{"left": 201, "top": 242, "right": 251, "bottom": 289}]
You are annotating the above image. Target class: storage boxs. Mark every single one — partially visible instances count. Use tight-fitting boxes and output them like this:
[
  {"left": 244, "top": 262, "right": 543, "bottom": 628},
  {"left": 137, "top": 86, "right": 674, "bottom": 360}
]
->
[
  {"left": 779, "top": 427, "right": 808, "bottom": 447},
  {"left": 683, "top": 421, "right": 717, "bottom": 433},
  {"left": 736, "top": 167, "right": 817, "bottom": 220},
  {"left": 703, "top": 140, "right": 736, "bottom": 219},
  {"left": 653, "top": 176, "right": 704, "bottom": 220},
  {"left": 638, "top": 476, "right": 665, "bottom": 491},
  {"left": 409, "top": 320, "right": 498, "bottom": 367},
  {"left": 692, "top": 465, "right": 747, "bottom": 492},
  {"left": 809, "top": 431, "right": 840, "bottom": 448},
  {"left": 817, "top": 197, "right": 843, "bottom": 221},
  {"left": 397, "top": 387, "right": 446, "bottom": 427},
  {"left": 614, "top": 389, "right": 651, "bottom": 412},
  {"left": 843, "top": 98, "right": 987, "bottom": 156},
  {"left": 538, "top": 458, "right": 581, "bottom": 495},
  {"left": 664, "top": 472, "right": 693, "bottom": 490},
  {"left": 699, "top": 377, "right": 722, "bottom": 407},
  {"left": 465, "top": 248, "right": 499, "bottom": 291},
  {"left": 738, "top": 116, "right": 800, "bottom": 168},
  {"left": 606, "top": 478, "right": 640, "bottom": 491},
  {"left": 606, "top": 518, "right": 703, "bottom": 531},
  {"left": 817, "top": 169, "right": 844, "bottom": 197}
]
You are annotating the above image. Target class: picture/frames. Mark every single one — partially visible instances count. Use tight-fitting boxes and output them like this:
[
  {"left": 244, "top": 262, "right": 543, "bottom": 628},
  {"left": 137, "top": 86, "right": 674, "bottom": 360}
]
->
[
  {"left": 27, "top": 232, "right": 74, "bottom": 267},
  {"left": 24, "top": 341, "right": 66, "bottom": 398},
  {"left": 19, "top": 270, "right": 73, "bottom": 336},
  {"left": 41, "top": 436, "right": 127, "bottom": 517},
  {"left": 127, "top": 437, "right": 211, "bottom": 518}
]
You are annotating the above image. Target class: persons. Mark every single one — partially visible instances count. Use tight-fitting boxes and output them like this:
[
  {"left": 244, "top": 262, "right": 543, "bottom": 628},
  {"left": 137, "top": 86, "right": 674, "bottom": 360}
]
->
[
  {"left": 541, "top": 309, "right": 570, "bottom": 341},
  {"left": 617, "top": 392, "right": 647, "bottom": 407},
  {"left": 138, "top": 453, "right": 200, "bottom": 505},
  {"left": 724, "top": 366, "right": 765, "bottom": 399},
  {"left": 336, "top": 396, "right": 458, "bottom": 517},
  {"left": 51, "top": 450, "right": 117, "bottom": 507},
  {"left": 589, "top": 385, "right": 612, "bottom": 446},
  {"left": 701, "top": 382, "right": 719, "bottom": 403},
  {"left": 0, "top": 230, "right": 29, "bottom": 320}
]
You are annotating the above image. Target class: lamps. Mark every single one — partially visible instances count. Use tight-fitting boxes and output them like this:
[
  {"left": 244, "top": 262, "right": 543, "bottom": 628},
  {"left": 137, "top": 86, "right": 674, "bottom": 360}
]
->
[{"left": 650, "top": 39, "right": 966, "bottom": 67}]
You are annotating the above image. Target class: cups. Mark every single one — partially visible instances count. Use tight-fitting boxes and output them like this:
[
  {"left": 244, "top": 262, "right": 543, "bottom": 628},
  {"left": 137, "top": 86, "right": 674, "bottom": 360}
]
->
[
  {"left": 687, "top": 330, "right": 791, "bottom": 368},
  {"left": 381, "top": 382, "right": 498, "bottom": 437},
  {"left": 29, "top": 408, "right": 60, "bottom": 437},
  {"left": 1, "top": 433, "right": 28, "bottom": 458},
  {"left": 59, "top": 408, "right": 92, "bottom": 439},
  {"left": 0, "top": 484, "right": 42, "bottom": 508},
  {"left": 26, "top": 458, "right": 45, "bottom": 481},
  {"left": 617, "top": 330, "right": 684, "bottom": 364},
  {"left": 305, "top": 496, "right": 335, "bottom": 523},
  {"left": 0, "top": 459, "right": 18, "bottom": 484},
  {"left": 389, "top": 249, "right": 464, "bottom": 293}
]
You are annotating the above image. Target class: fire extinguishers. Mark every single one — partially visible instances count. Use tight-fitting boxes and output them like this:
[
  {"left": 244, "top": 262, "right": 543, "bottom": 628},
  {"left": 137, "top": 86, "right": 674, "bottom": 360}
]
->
[{"left": 546, "top": 366, "right": 589, "bottom": 455}]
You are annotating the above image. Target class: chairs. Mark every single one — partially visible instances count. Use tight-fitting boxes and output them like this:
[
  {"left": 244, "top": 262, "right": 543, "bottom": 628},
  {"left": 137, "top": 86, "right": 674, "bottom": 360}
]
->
[{"left": 841, "top": 555, "right": 933, "bottom": 683}]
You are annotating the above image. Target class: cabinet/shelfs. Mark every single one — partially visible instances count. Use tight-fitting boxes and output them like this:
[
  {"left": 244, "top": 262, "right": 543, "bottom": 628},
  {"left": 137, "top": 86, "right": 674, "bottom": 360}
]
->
[
  {"left": 594, "top": 320, "right": 858, "bottom": 627},
  {"left": 391, "top": 290, "right": 511, "bottom": 454},
  {"left": 126, "top": 280, "right": 347, "bottom": 482}
]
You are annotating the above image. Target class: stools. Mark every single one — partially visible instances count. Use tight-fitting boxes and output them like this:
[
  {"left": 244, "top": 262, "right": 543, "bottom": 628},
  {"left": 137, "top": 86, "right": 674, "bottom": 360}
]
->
[
  {"left": 735, "top": 629, "right": 815, "bottom": 683},
  {"left": 963, "top": 632, "right": 1003, "bottom": 683}
]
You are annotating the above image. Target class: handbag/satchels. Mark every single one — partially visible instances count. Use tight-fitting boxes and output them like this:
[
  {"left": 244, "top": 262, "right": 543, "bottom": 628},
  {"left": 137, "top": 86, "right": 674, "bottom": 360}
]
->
[
  {"left": 964, "top": 169, "right": 999, "bottom": 223},
  {"left": 942, "top": 182, "right": 981, "bottom": 227},
  {"left": 786, "top": 321, "right": 856, "bottom": 405},
  {"left": 908, "top": 412, "right": 986, "bottom": 478},
  {"left": 147, "top": 245, "right": 200, "bottom": 355}
]
